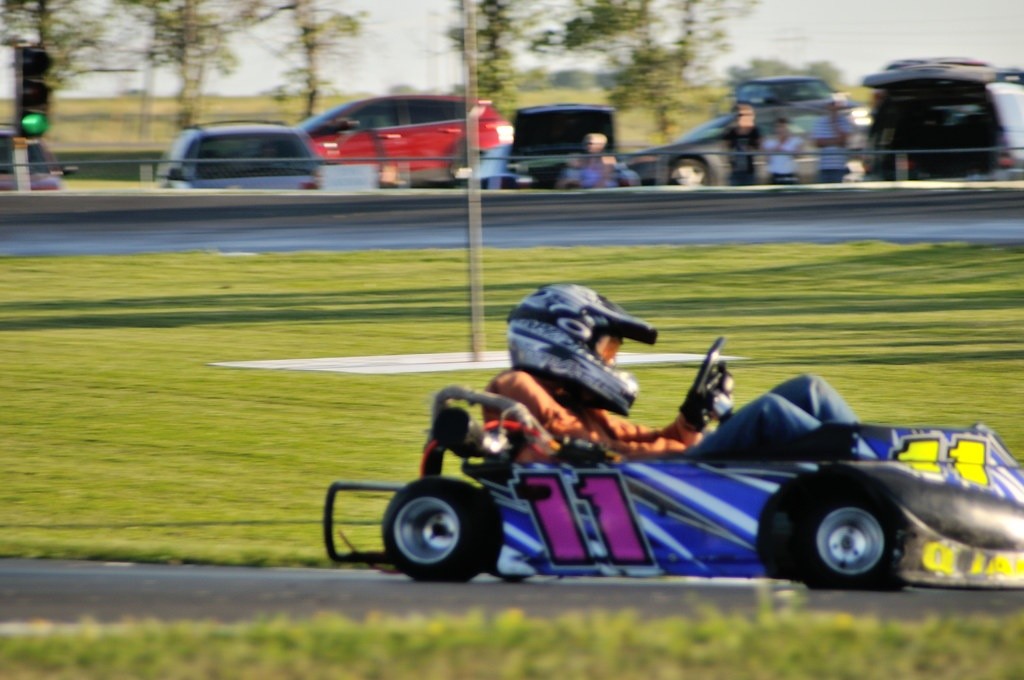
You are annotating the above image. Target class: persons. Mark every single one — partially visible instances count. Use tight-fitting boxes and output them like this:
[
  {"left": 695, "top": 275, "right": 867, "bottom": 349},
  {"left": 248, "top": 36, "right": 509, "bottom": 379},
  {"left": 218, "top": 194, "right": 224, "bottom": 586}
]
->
[
  {"left": 481, "top": 285, "right": 857, "bottom": 468},
  {"left": 811, "top": 100, "right": 853, "bottom": 183},
  {"left": 725, "top": 104, "right": 761, "bottom": 186},
  {"left": 989, "top": 153, "right": 1024, "bottom": 180},
  {"left": 761, "top": 117, "right": 802, "bottom": 186},
  {"left": 560, "top": 133, "right": 641, "bottom": 188}
]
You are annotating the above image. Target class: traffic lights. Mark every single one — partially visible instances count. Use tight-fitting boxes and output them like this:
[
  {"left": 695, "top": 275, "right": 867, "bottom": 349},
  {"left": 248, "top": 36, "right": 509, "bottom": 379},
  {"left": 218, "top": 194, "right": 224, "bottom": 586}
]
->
[{"left": 11, "top": 44, "right": 51, "bottom": 140}]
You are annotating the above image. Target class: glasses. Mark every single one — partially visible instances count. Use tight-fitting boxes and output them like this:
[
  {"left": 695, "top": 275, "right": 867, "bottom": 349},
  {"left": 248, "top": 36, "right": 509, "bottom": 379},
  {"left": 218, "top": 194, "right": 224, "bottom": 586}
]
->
[{"left": 585, "top": 328, "right": 622, "bottom": 363}]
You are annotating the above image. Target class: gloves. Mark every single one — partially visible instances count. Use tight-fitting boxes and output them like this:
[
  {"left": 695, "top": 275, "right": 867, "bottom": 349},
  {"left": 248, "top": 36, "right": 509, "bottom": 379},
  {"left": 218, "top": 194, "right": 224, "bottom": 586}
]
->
[{"left": 680, "top": 363, "right": 734, "bottom": 431}]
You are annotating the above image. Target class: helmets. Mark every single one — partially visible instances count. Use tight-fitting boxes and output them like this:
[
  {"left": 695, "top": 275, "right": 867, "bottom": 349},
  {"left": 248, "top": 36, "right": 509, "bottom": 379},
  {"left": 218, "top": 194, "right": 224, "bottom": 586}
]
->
[{"left": 507, "top": 282, "right": 657, "bottom": 418}]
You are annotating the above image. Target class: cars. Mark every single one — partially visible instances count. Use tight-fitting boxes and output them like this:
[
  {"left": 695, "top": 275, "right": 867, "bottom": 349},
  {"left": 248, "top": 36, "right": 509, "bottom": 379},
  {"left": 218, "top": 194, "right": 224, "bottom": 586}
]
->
[
  {"left": 0, "top": 123, "right": 79, "bottom": 191},
  {"left": 477, "top": 102, "right": 646, "bottom": 190},
  {"left": 621, "top": 52, "right": 1023, "bottom": 186},
  {"left": 155, "top": 119, "right": 326, "bottom": 191},
  {"left": 296, "top": 88, "right": 513, "bottom": 191}
]
